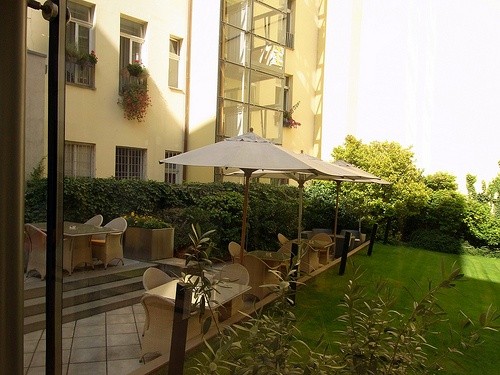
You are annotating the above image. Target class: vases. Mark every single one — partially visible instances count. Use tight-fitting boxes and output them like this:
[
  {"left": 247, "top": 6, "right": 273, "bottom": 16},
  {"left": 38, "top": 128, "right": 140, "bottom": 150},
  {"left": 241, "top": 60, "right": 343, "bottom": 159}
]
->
[{"left": 123, "top": 228, "right": 175, "bottom": 261}]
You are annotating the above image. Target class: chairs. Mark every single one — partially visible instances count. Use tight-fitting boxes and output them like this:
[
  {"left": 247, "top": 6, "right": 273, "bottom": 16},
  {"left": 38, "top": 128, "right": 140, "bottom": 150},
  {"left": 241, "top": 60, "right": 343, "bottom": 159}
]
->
[
  {"left": 139, "top": 233, "right": 365, "bottom": 363},
  {"left": 23, "top": 214, "right": 127, "bottom": 281}
]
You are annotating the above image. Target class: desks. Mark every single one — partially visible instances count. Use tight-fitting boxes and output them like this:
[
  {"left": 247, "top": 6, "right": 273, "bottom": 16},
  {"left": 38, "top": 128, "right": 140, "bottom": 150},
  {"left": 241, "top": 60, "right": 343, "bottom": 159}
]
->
[
  {"left": 33, "top": 221, "right": 122, "bottom": 276},
  {"left": 291, "top": 239, "right": 333, "bottom": 248},
  {"left": 149, "top": 273, "right": 251, "bottom": 311},
  {"left": 332, "top": 235, "right": 353, "bottom": 240},
  {"left": 249, "top": 250, "right": 291, "bottom": 262}
]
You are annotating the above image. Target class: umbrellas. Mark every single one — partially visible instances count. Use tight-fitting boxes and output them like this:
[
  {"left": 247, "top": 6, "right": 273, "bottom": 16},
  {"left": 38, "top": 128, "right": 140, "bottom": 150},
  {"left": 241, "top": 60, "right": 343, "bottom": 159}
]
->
[
  {"left": 310, "top": 157, "right": 393, "bottom": 239},
  {"left": 159, "top": 128, "right": 331, "bottom": 265},
  {"left": 226, "top": 150, "right": 381, "bottom": 257}
]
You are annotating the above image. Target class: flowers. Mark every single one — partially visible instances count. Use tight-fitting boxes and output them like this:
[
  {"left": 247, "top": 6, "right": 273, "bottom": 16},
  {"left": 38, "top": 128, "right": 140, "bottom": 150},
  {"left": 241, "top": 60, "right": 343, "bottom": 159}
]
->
[
  {"left": 127, "top": 60, "right": 148, "bottom": 77},
  {"left": 123, "top": 212, "right": 171, "bottom": 230},
  {"left": 117, "top": 81, "right": 152, "bottom": 123},
  {"left": 284, "top": 110, "right": 301, "bottom": 129},
  {"left": 78, "top": 50, "right": 99, "bottom": 66}
]
